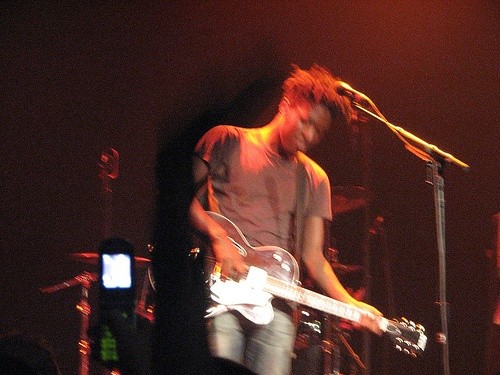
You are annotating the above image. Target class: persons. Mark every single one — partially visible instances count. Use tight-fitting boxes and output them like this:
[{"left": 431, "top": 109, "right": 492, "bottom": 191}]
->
[
  {"left": 186, "top": 61, "right": 384, "bottom": 375},
  {"left": 0, "top": 289, "right": 144, "bottom": 374}
]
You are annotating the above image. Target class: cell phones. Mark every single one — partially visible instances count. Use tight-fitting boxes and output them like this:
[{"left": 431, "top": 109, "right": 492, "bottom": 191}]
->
[{"left": 97, "top": 237, "right": 135, "bottom": 369}]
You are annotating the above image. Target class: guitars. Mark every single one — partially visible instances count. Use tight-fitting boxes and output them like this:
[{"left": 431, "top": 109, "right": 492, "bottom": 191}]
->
[{"left": 204, "top": 210, "right": 428, "bottom": 358}]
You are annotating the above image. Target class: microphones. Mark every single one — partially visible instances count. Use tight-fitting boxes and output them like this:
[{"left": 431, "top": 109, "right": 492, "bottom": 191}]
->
[{"left": 332, "top": 81, "right": 372, "bottom": 107}]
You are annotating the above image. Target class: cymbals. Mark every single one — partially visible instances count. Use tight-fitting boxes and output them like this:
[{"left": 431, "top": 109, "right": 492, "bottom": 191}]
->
[{"left": 331, "top": 185, "right": 368, "bottom": 214}]
